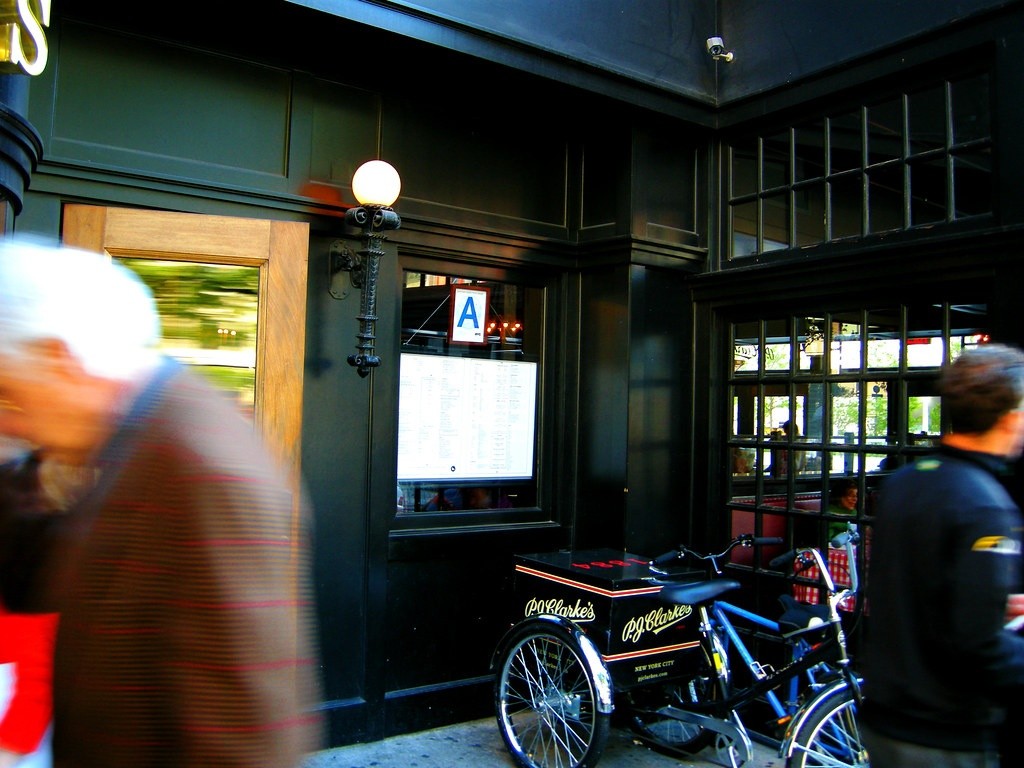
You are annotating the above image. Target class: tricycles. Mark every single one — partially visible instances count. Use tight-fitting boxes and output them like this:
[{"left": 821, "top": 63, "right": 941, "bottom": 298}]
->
[{"left": 492, "top": 528, "right": 872, "bottom": 768}]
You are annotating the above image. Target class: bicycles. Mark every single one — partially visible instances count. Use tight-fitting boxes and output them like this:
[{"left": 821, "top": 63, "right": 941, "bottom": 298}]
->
[{"left": 653, "top": 533, "right": 872, "bottom": 768}]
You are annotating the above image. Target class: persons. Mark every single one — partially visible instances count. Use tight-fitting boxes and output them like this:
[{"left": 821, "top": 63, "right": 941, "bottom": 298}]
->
[
  {"left": 782, "top": 420, "right": 807, "bottom": 472},
  {"left": 823, "top": 479, "right": 858, "bottom": 541},
  {"left": 0, "top": 234, "right": 319, "bottom": 768},
  {"left": 852, "top": 345, "right": 1024, "bottom": 768}
]
